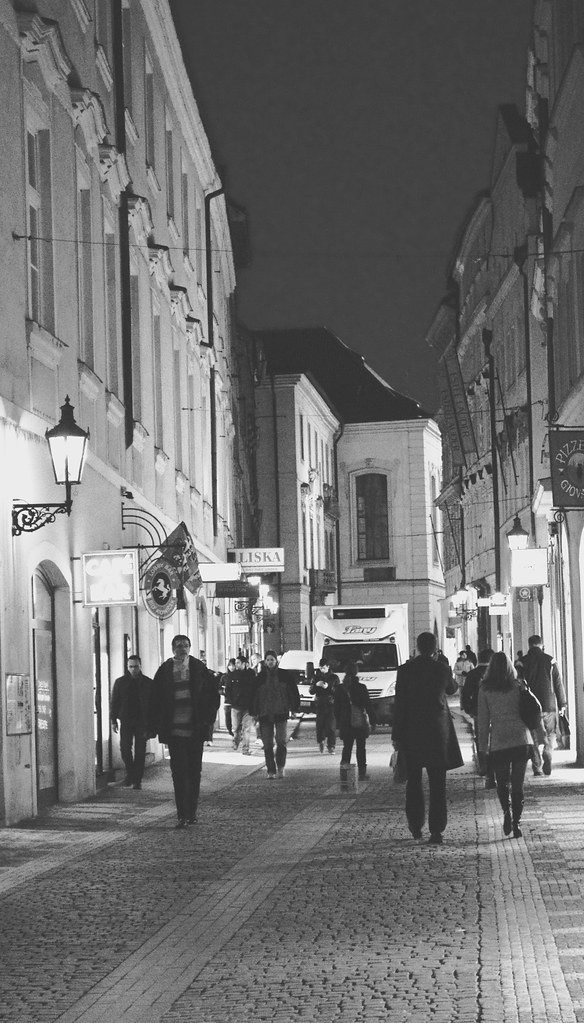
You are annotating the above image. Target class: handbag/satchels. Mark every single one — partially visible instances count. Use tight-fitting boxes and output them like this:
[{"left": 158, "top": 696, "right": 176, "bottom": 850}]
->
[
  {"left": 517, "top": 681, "right": 542, "bottom": 730},
  {"left": 559, "top": 712, "right": 570, "bottom": 736},
  {"left": 390, "top": 749, "right": 405, "bottom": 783},
  {"left": 350, "top": 704, "right": 370, "bottom": 738}
]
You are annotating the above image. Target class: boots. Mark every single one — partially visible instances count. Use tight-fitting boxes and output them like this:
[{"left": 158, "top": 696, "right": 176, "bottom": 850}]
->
[{"left": 358, "top": 764, "right": 370, "bottom": 781}]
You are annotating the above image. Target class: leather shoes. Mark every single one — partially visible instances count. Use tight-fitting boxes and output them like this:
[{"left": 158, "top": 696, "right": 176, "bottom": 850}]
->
[
  {"left": 176, "top": 817, "right": 189, "bottom": 829},
  {"left": 190, "top": 814, "right": 197, "bottom": 823}
]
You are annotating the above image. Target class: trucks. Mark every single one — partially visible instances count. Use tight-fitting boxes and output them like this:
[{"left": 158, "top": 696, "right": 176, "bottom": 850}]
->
[{"left": 306, "top": 603, "right": 410, "bottom": 730}]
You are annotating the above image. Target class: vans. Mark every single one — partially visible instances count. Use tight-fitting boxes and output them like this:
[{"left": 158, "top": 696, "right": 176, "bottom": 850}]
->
[{"left": 276, "top": 649, "right": 322, "bottom": 719}]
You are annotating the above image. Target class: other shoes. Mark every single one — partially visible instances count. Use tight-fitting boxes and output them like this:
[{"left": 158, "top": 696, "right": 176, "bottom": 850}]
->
[
  {"left": 543, "top": 751, "right": 551, "bottom": 775},
  {"left": 486, "top": 779, "right": 497, "bottom": 788},
  {"left": 513, "top": 828, "right": 522, "bottom": 838},
  {"left": 266, "top": 773, "right": 275, "bottom": 778},
  {"left": 413, "top": 831, "right": 422, "bottom": 840},
  {"left": 534, "top": 771, "right": 543, "bottom": 776},
  {"left": 504, "top": 811, "right": 512, "bottom": 835},
  {"left": 132, "top": 780, "right": 141, "bottom": 790},
  {"left": 277, "top": 764, "right": 285, "bottom": 778},
  {"left": 429, "top": 833, "right": 442, "bottom": 843}
]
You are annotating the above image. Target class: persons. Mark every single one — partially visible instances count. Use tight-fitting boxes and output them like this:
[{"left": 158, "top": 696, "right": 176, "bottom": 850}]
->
[
  {"left": 308, "top": 658, "right": 341, "bottom": 755},
  {"left": 478, "top": 652, "right": 542, "bottom": 838},
  {"left": 154, "top": 635, "right": 221, "bottom": 828},
  {"left": 334, "top": 663, "right": 375, "bottom": 769},
  {"left": 391, "top": 633, "right": 465, "bottom": 842},
  {"left": 253, "top": 650, "right": 300, "bottom": 779},
  {"left": 517, "top": 634, "right": 567, "bottom": 778},
  {"left": 108, "top": 655, "right": 154, "bottom": 789},
  {"left": 432, "top": 643, "right": 526, "bottom": 789},
  {"left": 201, "top": 654, "right": 275, "bottom": 756}
]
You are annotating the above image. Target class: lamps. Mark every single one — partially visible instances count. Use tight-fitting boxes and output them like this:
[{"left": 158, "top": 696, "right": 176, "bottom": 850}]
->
[
  {"left": 235, "top": 573, "right": 279, "bottom": 624},
  {"left": 451, "top": 584, "right": 481, "bottom": 621},
  {"left": 11, "top": 392, "right": 91, "bottom": 538},
  {"left": 506, "top": 513, "right": 550, "bottom": 587}
]
